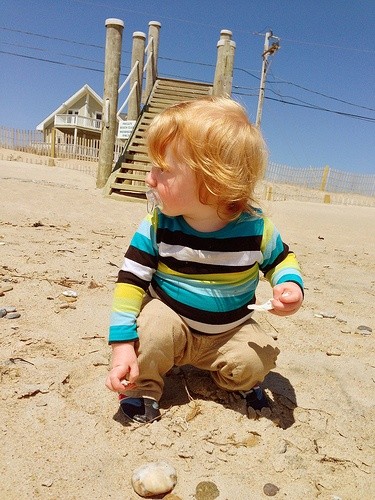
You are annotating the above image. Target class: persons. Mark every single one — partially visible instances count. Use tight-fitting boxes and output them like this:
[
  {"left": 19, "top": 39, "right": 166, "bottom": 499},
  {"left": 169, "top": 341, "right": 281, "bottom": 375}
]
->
[{"left": 101, "top": 93, "right": 306, "bottom": 425}]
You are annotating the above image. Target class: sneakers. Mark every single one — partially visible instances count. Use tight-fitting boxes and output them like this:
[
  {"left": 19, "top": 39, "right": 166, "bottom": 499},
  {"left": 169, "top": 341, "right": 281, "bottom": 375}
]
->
[
  {"left": 118, "top": 391, "right": 161, "bottom": 425},
  {"left": 236, "top": 384, "right": 273, "bottom": 411}
]
